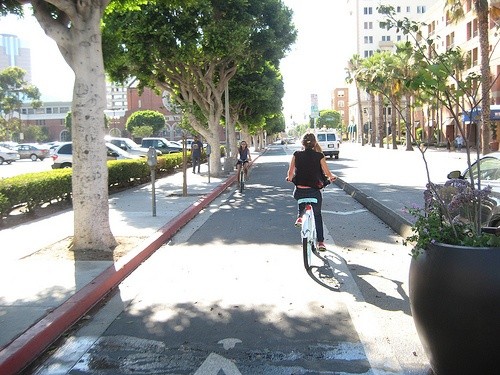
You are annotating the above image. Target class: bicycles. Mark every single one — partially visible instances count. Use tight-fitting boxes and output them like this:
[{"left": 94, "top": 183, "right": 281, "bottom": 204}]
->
[
  {"left": 286, "top": 177, "right": 335, "bottom": 268},
  {"left": 234, "top": 159, "right": 249, "bottom": 192}
]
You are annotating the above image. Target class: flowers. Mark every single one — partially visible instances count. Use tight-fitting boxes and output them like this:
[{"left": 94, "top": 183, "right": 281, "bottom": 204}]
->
[{"left": 343, "top": 1, "right": 500, "bottom": 258}]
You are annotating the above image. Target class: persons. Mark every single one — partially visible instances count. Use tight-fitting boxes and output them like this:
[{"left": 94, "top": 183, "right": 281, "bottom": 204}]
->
[
  {"left": 447, "top": 136, "right": 451, "bottom": 150},
  {"left": 191, "top": 136, "right": 203, "bottom": 174},
  {"left": 288, "top": 133, "right": 336, "bottom": 251},
  {"left": 455, "top": 135, "right": 462, "bottom": 151},
  {"left": 235, "top": 141, "right": 251, "bottom": 187}
]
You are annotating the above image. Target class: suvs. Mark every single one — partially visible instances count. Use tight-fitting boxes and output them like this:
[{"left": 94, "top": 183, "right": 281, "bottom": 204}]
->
[
  {"left": 104, "top": 137, "right": 162, "bottom": 159},
  {"left": 140, "top": 138, "right": 182, "bottom": 155},
  {"left": 50, "top": 140, "right": 138, "bottom": 169}
]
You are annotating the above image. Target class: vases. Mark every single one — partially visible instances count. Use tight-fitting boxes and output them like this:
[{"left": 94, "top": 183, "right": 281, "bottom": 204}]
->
[{"left": 409, "top": 238, "right": 500, "bottom": 375}]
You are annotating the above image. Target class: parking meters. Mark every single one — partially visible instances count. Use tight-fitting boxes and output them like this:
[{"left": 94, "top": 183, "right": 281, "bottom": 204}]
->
[{"left": 148, "top": 146, "right": 157, "bottom": 216}]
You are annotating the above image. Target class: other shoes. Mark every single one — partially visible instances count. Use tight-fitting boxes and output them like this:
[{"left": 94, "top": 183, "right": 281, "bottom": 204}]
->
[
  {"left": 318, "top": 242, "right": 326, "bottom": 251},
  {"left": 295, "top": 218, "right": 301, "bottom": 227},
  {"left": 192, "top": 172, "right": 195, "bottom": 174}
]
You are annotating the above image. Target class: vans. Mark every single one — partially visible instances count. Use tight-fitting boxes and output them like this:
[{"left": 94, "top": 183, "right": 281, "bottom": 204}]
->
[{"left": 314, "top": 132, "right": 340, "bottom": 159}]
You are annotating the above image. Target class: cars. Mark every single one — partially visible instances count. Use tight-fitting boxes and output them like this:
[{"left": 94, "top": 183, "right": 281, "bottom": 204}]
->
[
  {"left": 0, "top": 141, "right": 60, "bottom": 154},
  {"left": 281, "top": 138, "right": 295, "bottom": 145},
  {"left": 170, "top": 139, "right": 207, "bottom": 150},
  {"left": 444, "top": 152, "right": 500, "bottom": 229},
  {"left": 0, "top": 146, "right": 20, "bottom": 165},
  {"left": 8, "top": 144, "right": 50, "bottom": 162}
]
